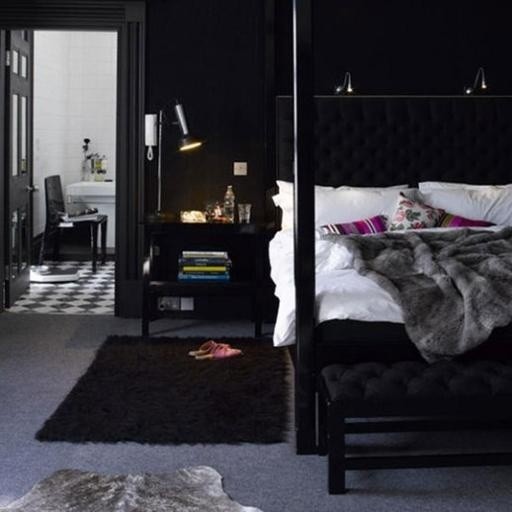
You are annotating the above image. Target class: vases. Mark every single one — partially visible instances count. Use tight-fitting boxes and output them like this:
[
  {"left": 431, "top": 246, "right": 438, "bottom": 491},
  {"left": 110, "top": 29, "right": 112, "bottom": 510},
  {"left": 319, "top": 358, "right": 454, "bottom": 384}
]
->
[{"left": 80, "top": 160, "right": 90, "bottom": 181}]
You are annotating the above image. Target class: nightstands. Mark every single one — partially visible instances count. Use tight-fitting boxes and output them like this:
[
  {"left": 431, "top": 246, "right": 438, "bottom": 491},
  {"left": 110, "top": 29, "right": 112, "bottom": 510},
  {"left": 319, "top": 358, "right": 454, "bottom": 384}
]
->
[{"left": 138, "top": 212, "right": 282, "bottom": 337}]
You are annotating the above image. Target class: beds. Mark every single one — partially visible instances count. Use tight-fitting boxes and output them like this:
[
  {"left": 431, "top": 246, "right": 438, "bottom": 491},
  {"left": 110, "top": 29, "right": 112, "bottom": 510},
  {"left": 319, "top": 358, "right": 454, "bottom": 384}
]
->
[{"left": 268, "top": 2, "right": 512, "bottom": 456}]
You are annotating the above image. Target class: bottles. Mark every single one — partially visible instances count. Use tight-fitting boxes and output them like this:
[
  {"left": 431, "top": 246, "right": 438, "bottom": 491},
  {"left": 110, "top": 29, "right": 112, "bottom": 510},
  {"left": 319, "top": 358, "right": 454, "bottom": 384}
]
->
[{"left": 224, "top": 185, "right": 235, "bottom": 222}]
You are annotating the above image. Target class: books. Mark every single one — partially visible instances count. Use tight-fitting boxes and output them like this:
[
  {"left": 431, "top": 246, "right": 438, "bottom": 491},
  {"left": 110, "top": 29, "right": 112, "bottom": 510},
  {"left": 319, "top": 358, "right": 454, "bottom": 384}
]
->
[{"left": 177, "top": 249, "right": 234, "bottom": 280}]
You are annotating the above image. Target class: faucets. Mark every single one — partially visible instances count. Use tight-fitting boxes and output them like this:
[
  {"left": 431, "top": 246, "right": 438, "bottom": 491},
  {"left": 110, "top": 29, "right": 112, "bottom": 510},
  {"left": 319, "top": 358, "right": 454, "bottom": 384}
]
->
[{"left": 81, "top": 154, "right": 99, "bottom": 181}]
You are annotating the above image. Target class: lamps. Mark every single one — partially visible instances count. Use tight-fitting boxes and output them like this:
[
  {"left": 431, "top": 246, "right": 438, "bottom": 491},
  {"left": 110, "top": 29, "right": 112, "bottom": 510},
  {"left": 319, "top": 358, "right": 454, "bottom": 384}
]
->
[{"left": 144, "top": 104, "right": 203, "bottom": 217}]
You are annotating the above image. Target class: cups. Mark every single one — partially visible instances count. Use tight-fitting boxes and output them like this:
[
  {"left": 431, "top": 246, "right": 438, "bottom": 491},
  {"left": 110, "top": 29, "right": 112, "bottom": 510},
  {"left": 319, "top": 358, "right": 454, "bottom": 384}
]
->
[{"left": 237, "top": 204, "right": 251, "bottom": 223}]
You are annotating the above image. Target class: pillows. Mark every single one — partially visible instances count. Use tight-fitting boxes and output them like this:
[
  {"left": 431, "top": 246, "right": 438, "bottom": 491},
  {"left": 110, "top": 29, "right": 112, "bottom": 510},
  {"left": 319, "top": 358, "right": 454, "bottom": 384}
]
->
[{"left": 275, "top": 179, "right": 512, "bottom": 240}]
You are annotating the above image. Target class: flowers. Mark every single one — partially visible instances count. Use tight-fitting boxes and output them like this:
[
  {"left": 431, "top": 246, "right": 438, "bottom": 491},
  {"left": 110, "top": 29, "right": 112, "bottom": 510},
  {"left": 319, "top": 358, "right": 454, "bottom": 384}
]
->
[{"left": 82, "top": 139, "right": 92, "bottom": 162}]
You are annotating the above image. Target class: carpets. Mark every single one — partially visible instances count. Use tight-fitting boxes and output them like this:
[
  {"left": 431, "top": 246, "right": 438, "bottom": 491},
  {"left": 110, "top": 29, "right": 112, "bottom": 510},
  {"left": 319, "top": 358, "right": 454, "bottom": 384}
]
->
[
  {"left": 35, "top": 334, "right": 292, "bottom": 446},
  {"left": 0, "top": 464, "right": 264, "bottom": 512}
]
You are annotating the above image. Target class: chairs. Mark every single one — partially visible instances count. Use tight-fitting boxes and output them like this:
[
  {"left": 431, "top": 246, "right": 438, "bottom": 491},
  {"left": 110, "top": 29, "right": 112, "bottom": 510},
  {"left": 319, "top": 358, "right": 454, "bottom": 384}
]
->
[{"left": 39, "top": 175, "right": 108, "bottom": 274}]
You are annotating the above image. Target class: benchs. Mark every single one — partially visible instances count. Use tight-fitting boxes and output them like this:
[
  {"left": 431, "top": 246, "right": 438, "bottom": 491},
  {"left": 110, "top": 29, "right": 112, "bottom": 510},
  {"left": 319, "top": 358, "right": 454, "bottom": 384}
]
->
[{"left": 305, "top": 355, "right": 512, "bottom": 495}]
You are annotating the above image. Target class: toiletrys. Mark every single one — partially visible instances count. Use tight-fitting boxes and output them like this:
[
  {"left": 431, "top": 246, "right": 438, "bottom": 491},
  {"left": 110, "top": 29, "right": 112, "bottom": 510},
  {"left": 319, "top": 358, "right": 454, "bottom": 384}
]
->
[{"left": 88, "top": 153, "right": 113, "bottom": 183}]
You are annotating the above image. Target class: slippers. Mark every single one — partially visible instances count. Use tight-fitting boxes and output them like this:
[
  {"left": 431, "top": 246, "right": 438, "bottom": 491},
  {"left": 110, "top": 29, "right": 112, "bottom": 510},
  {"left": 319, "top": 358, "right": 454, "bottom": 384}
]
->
[{"left": 188, "top": 340, "right": 241, "bottom": 361}]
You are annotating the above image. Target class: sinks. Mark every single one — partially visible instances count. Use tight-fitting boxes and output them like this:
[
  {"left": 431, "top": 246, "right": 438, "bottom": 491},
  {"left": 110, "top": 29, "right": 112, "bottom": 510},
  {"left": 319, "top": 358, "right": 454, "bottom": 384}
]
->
[{"left": 67, "top": 182, "right": 116, "bottom": 196}]
